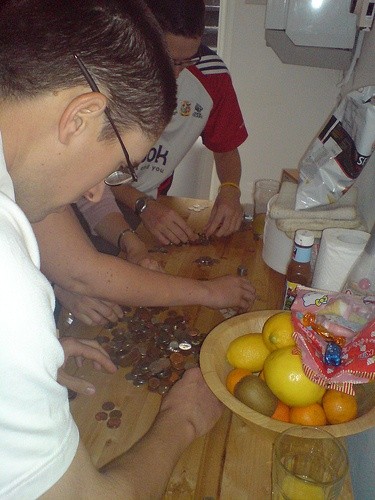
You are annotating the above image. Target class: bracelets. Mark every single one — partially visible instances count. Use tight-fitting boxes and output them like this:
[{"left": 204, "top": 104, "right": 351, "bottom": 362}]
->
[
  {"left": 218, "top": 182, "right": 242, "bottom": 199},
  {"left": 117, "top": 229, "right": 138, "bottom": 253}
]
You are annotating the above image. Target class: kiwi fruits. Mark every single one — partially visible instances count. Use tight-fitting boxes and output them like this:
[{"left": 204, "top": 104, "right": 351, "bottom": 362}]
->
[{"left": 233, "top": 375, "right": 278, "bottom": 417}]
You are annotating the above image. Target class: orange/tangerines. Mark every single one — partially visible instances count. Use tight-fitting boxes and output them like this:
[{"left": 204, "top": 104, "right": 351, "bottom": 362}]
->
[{"left": 226, "top": 368, "right": 357, "bottom": 426}]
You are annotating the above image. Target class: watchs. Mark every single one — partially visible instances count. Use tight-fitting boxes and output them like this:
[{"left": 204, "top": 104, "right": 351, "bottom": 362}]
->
[{"left": 135, "top": 196, "right": 155, "bottom": 215}]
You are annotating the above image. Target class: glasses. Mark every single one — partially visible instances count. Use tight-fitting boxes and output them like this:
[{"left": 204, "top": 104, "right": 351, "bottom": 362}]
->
[
  {"left": 67, "top": 53, "right": 139, "bottom": 186},
  {"left": 169, "top": 51, "right": 202, "bottom": 70}
]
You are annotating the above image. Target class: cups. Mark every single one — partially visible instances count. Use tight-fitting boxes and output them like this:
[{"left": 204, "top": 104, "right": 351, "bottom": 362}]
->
[
  {"left": 270, "top": 425, "right": 349, "bottom": 500},
  {"left": 254, "top": 179, "right": 279, "bottom": 235}
]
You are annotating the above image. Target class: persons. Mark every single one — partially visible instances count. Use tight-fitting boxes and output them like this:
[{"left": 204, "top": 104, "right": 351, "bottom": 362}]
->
[
  {"left": 0, "top": 0, "right": 225, "bottom": 500},
  {"left": 50, "top": 180, "right": 166, "bottom": 332},
  {"left": 110, "top": 0, "right": 249, "bottom": 246},
  {"left": 30, "top": 206, "right": 257, "bottom": 311}
]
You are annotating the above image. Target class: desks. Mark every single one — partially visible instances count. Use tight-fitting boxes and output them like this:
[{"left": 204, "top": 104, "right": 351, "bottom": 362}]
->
[{"left": 61, "top": 193, "right": 353, "bottom": 500}]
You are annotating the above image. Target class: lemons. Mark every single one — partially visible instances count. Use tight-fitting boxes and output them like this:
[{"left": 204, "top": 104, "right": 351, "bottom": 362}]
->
[
  {"left": 264, "top": 345, "right": 328, "bottom": 406},
  {"left": 262, "top": 311, "right": 298, "bottom": 351},
  {"left": 227, "top": 333, "right": 272, "bottom": 373}
]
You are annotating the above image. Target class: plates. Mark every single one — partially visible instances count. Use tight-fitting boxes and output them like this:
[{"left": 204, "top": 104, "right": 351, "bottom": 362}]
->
[{"left": 199, "top": 310, "right": 375, "bottom": 437}]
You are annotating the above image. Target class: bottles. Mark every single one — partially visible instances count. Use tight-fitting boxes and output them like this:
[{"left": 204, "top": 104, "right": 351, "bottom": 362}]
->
[
  {"left": 283, "top": 230, "right": 313, "bottom": 309},
  {"left": 340, "top": 223, "right": 375, "bottom": 304}
]
